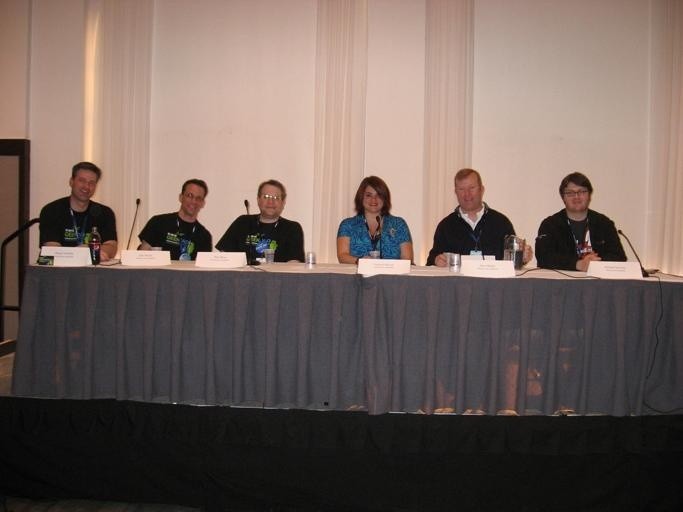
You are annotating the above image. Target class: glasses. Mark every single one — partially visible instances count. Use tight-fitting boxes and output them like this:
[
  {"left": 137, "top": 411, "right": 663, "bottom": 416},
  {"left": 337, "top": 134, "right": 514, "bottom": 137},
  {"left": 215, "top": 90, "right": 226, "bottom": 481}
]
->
[
  {"left": 264, "top": 195, "right": 280, "bottom": 200},
  {"left": 565, "top": 191, "right": 588, "bottom": 197}
]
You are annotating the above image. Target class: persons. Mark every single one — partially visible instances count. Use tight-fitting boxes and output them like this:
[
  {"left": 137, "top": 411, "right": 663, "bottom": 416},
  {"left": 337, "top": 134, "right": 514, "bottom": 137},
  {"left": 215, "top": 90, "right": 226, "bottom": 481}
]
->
[
  {"left": 425, "top": 167, "right": 531, "bottom": 267},
  {"left": 215, "top": 180, "right": 306, "bottom": 266},
  {"left": 336, "top": 177, "right": 413, "bottom": 266},
  {"left": 135, "top": 177, "right": 211, "bottom": 262},
  {"left": 38, "top": 160, "right": 116, "bottom": 262},
  {"left": 534, "top": 172, "right": 627, "bottom": 271}
]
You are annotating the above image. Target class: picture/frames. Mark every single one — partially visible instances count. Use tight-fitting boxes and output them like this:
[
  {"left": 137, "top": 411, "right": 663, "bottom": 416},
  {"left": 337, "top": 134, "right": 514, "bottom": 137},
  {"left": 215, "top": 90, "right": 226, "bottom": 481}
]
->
[{"left": 0, "top": 138, "right": 31, "bottom": 361}]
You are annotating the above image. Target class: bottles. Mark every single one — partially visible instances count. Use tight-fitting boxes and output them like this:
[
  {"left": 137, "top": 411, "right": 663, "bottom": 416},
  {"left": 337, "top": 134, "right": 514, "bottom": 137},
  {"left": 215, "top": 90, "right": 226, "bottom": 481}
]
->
[
  {"left": 86, "top": 227, "right": 101, "bottom": 264},
  {"left": 506, "top": 235, "right": 522, "bottom": 272}
]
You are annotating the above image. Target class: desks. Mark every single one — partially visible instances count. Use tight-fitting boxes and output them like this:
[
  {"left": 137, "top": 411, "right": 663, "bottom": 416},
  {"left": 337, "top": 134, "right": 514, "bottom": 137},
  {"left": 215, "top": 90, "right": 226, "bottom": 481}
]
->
[{"left": 10, "top": 262, "right": 683, "bottom": 417}]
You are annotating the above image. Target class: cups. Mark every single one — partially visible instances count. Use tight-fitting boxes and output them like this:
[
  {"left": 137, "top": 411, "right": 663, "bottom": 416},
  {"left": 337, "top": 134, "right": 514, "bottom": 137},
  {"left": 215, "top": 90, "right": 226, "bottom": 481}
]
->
[
  {"left": 305, "top": 252, "right": 314, "bottom": 265},
  {"left": 450, "top": 252, "right": 460, "bottom": 272},
  {"left": 263, "top": 247, "right": 273, "bottom": 264}
]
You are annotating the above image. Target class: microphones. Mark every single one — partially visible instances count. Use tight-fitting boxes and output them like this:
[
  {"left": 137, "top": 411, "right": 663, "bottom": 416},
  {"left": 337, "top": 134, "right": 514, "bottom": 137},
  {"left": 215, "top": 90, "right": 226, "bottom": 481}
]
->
[
  {"left": 244, "top": 200, "right": 261, "bottom": 265},
  {"left": 376, "top": 216, "right": 382, "bottom": 259},
  {"left": 618, "top": 230, "right": 648, "bottom": 277},
  {"left": 127, "top": 199, "right": 140, "bottom": 250}
]
